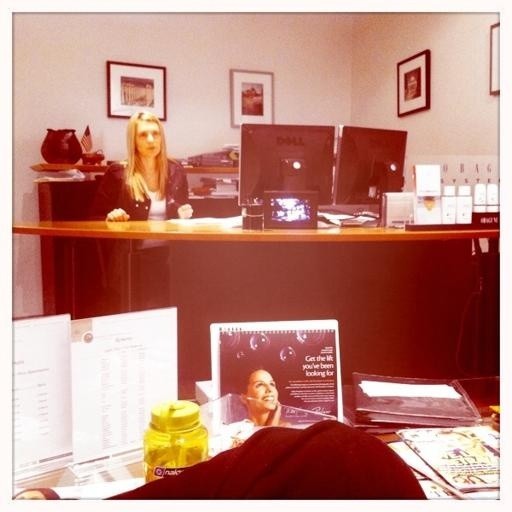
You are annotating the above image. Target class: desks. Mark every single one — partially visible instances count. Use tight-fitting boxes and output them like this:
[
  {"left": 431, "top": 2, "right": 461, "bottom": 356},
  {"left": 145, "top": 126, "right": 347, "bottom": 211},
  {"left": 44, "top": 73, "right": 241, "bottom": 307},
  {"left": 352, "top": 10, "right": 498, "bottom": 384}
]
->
[{"left": 104, "top": 355, "right": 499, "bottom": 498}]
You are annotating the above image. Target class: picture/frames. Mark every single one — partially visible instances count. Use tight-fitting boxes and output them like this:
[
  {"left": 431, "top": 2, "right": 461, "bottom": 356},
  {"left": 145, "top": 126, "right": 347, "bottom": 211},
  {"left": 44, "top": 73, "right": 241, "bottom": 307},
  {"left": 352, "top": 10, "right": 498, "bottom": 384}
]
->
[
  {"left": 105, "top": 60, "right": 168, "bottom": 122},
  {"left": 395, "top": 47, "right": 430, "bottom": 117},
  {"left": 230, "top": 68, "right": 276, "bottom": 128}
]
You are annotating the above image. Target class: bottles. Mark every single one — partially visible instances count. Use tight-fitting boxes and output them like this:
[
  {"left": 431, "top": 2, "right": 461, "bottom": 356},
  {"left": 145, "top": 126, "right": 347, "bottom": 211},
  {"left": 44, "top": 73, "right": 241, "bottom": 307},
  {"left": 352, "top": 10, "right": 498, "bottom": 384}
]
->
[
  {"left": 440, "top": 183, "right": 498, "bottom": 225},
  {"left": 41, "top": 129, "right": 83, "bottom": 165}
]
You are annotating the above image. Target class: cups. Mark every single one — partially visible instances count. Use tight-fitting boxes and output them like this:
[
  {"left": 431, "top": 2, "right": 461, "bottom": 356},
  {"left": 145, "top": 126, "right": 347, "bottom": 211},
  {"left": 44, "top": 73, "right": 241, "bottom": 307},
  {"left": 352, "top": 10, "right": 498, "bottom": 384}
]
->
[{"left": 143, "top": 399, "right": 209, "bottom": 484}]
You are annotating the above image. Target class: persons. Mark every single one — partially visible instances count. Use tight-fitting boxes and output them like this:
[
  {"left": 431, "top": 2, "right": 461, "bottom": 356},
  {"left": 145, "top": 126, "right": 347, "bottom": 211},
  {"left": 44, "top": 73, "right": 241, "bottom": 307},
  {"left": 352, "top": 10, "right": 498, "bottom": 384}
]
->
[
  {"left": 82, "top": 111, "right": 194, "bottom": 316},
  {"left": 226, "top": 367, "right": 292, "bottom": 448}
]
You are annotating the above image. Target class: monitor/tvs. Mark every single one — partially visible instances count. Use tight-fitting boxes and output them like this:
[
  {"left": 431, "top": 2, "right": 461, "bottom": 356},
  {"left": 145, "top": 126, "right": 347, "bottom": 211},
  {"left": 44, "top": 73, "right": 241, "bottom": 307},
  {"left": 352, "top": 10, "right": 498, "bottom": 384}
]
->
[
  {"left": 238, "top": 122, "right": 335, "bottom": 207},
  {"left": 331, "top": 125, "right": 408, "bottom": 205}
]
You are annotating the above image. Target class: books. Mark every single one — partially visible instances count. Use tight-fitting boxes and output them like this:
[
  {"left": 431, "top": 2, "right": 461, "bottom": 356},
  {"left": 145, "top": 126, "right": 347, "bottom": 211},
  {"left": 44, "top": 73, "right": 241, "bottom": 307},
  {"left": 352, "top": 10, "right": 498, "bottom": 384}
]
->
[{"left": 342, "top": 371, "right": 500, "bottom": 500}]
